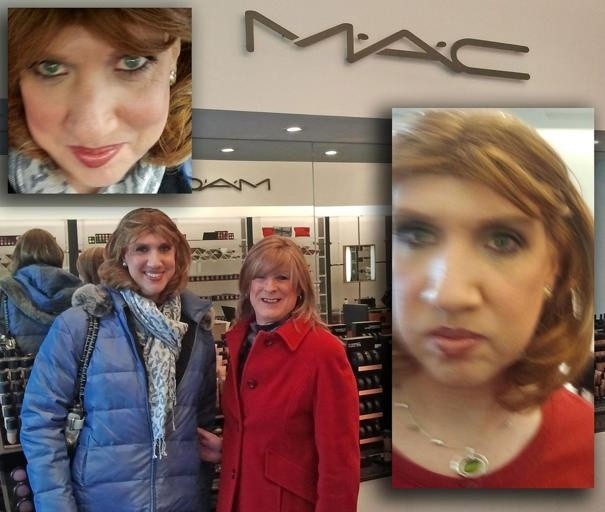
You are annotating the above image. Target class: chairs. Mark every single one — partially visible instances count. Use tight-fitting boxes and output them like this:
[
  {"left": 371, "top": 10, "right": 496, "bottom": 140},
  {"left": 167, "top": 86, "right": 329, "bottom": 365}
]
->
[{"left": 341, "top": 304, "right": 369, "bottom": 322}]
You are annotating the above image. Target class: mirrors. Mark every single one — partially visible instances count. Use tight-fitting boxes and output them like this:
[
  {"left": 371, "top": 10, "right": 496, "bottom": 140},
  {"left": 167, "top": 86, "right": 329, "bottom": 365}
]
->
[{"left": 343, "top": 244, "right": 376, "bottom": 282}]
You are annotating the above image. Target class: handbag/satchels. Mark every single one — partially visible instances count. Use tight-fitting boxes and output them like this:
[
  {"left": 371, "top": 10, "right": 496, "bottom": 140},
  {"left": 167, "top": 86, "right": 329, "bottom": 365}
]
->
[
  {"left": 65, "top": 404, "right": 84, "bottom": 446},
  {"left": 1, "top": 335, "right": 16, "bottom": 351}
]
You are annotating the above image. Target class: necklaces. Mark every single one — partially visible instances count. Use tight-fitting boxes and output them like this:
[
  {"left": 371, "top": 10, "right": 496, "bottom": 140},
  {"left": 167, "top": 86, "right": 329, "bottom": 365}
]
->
[{"left": 401, "top": 400, "right": 499, "bottom": 478}]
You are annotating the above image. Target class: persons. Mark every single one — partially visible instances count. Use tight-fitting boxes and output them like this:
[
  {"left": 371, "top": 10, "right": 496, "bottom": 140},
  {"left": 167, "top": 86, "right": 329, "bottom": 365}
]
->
[
  {"left": 0, "top": 229, "right": 84, "bottom": 352},
  {"left": 8, "top": 8, "right": 192, "bottom": 194},
  {"left": 197, "top": 236, "right": 361, "bottom": 512},
  {"left": 77, "top": 247, "right": 108, "bottom": 285},
  {"left": 392, "top": 105, "right": 594, "bottom": 488},
  {"left": 20, "top": 208, "right": 217, "bottom": 512}
]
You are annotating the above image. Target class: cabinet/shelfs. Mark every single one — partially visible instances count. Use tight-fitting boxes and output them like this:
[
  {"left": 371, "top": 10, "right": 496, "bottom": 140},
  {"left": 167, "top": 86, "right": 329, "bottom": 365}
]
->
[
  {"left": 247, "top": 214, "right": 329, "bottom": 326},
  {"left": 0, "top": 356, "right": 38, "bottom": 512},
  {"left": 337, "top": 322, "right": 391, "bottom": 481},
  {"left": 68, "top": 219, "right": 254, "bottom": 512},
  {"left": 595, "top": 314, "right": 604, "bottom": 434}
]
